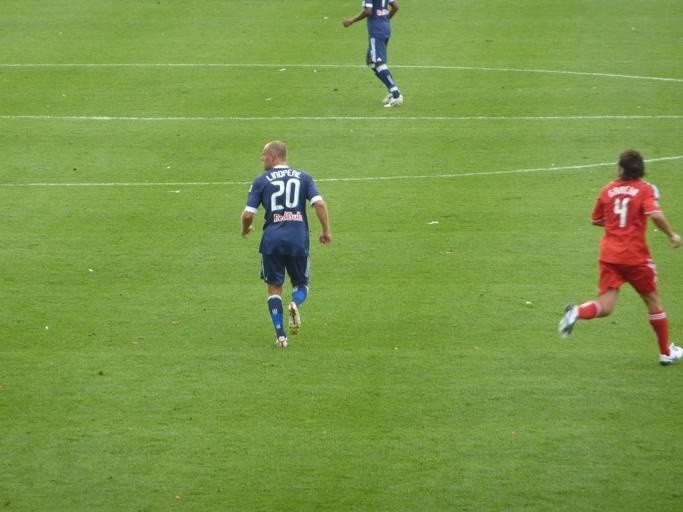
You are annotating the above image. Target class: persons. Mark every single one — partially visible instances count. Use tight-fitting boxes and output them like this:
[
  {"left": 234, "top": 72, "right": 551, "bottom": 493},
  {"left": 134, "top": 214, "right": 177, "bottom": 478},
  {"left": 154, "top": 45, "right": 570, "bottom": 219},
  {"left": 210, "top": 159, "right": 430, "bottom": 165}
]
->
[
  {"left": 239, "top": 140, "right": 334, "bottom": 349},
  {"left": 557, "top": 148, "right": 683, "bottom": 367},
  {"left": 342, "top": 0, "right": 404, "bottom": 107}
]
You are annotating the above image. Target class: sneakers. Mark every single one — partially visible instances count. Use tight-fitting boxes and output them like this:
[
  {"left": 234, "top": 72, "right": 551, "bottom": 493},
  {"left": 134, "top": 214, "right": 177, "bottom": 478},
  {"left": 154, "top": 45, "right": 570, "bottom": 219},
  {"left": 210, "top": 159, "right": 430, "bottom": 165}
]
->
[
  {"left": 659, "top": 343, "right": 683, "bottom": 365},
  {"left": 384, "top": 94, "right": 403, "bottom": 107},
  {"left": 288, "top": 302, "right": 301, "bottom": 334},
  {"left": 558, "top": 304, "right": 578, "bottom": 338},
  {"left": 276, "top": 336, "right": 288, "bottom": 347}
]
